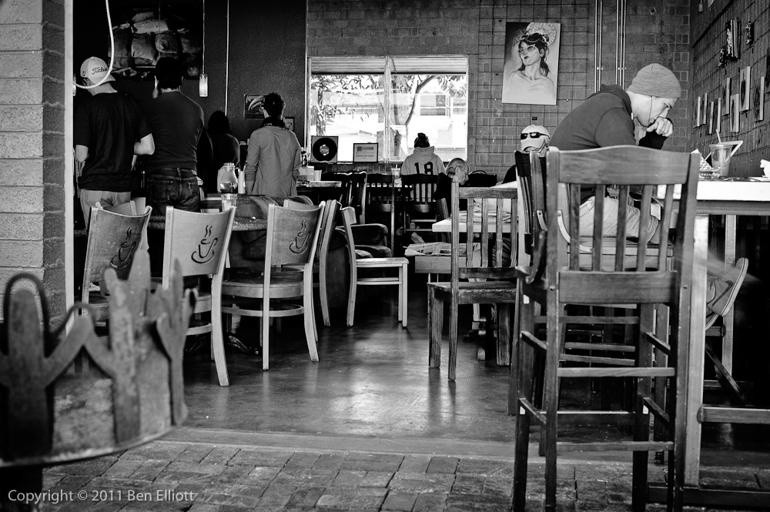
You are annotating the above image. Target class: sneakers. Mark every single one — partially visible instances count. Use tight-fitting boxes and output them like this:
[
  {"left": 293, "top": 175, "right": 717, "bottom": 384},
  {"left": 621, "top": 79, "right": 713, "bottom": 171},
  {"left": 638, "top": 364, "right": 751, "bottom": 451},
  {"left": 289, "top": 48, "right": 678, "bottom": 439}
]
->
[
  {"left": 707, "top": 257, "right": 749, "bottom": 318},
  {"left": 706, "top": 314, "right": 718, "bottom": 330}
]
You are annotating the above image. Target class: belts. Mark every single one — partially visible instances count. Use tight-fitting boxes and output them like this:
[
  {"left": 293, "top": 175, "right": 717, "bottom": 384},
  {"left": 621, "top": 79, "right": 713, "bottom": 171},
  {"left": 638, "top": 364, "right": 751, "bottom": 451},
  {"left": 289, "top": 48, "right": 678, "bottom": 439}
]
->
[{"left": 152, "top": 169, "right": 197, "bottom": 178}]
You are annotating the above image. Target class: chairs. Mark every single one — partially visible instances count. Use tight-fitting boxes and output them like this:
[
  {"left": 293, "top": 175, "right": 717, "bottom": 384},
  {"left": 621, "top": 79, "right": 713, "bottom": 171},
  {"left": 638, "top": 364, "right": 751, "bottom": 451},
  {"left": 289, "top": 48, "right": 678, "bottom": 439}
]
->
[{"left": 2, "top": 139, "right": 770, "bottom": 512}]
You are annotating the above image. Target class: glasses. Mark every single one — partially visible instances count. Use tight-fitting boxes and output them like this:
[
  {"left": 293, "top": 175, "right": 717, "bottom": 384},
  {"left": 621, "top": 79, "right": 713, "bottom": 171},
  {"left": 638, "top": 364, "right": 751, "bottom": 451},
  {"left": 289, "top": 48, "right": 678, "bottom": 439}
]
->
[
  {"left": 521, "top": 131, "right": 544, "bottom": 139},
  {"left": 519, "top": 45, "right": 535, "bottom": 55}
]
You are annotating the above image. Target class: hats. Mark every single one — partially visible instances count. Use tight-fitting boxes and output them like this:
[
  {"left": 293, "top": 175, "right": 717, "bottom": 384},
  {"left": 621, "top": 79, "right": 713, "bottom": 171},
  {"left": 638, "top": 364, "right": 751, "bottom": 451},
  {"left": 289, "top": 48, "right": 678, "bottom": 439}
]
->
[
  {"left": 414, "top": 133, "right": 430, "bottom": 148},
  {"left": 628, "top": 63, "right": 681, "bottom": 99},
  {"left": 79, "top": 56, "right": 116, "bottom": 86},
  {"left": 520, "top": 124, "right": 550, "bottom": 153}
]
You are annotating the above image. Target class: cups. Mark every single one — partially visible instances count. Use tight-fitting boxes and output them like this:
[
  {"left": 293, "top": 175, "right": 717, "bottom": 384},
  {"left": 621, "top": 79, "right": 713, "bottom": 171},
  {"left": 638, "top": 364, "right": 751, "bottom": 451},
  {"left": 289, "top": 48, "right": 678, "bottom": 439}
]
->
[
  {"left": 390, "top": 168, "right": 401, "bottom": 183},
  {"left": 708, "top": 143, "right": 733, "bottom": 176},
  {"left": 306, "top": 166, "right": 323, "bottom": 181}
]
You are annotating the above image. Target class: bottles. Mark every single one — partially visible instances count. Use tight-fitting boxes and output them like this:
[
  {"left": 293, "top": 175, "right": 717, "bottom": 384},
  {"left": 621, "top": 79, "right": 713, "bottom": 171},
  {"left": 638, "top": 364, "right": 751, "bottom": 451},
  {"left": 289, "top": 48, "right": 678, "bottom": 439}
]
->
[{"left": 219, "top": 162, "right": 238, "bottom": 223}]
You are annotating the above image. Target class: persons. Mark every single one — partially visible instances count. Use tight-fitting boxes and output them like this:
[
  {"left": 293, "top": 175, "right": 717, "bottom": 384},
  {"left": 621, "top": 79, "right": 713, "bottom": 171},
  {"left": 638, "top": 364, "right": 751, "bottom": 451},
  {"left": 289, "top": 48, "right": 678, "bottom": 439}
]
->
[
  {"left": 548, "top": 64, "right": 748, "bottom": 332},
  {"left": 203, "top": 111, "right": 241, "bottom": 194},
  {"left": 147, "top": 59, "right": 210, "bottom": 379},
  {"left": 74, "top": 57, "right": 155, "bottom": 289},
  {"left": 244, "top": 94, "right": 301, "bottom": 198},
  {"left": 509, "top": 34, "right": 554, "bottom": 91},
  {"left": 435, "top": 159, "right": 470, "bottom": 243},
  {"left": 502, "top": 122, "right": 552, "bottom": 286},
  {"left": 401, "top": 133, "right": 446, "bottom": 200}
]
